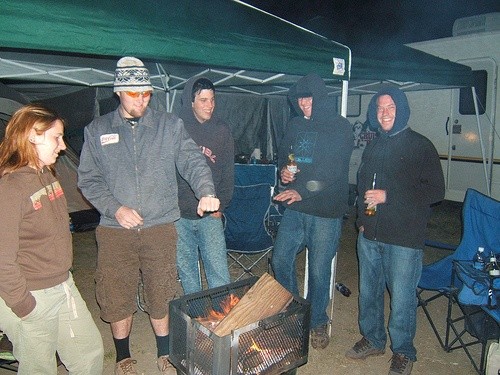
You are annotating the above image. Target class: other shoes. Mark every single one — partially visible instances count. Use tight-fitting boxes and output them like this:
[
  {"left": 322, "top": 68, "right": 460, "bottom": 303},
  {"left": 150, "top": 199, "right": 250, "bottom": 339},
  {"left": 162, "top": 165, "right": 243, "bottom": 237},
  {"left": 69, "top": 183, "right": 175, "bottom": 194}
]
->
[
  {"left": 114, "top": 358, "right": 139, "bottom": 375},
  {"left": 156, "top": 355, "right": 177, "bottom": 375}
]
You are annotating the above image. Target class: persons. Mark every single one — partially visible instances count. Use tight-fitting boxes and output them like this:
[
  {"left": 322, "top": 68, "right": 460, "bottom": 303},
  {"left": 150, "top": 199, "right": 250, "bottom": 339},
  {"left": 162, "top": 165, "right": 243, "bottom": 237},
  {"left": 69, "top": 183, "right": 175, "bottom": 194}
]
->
[
  {"left": 270, "top": 74, "right": 355, "bottom": 350},
  {"left": 344, "top": 84, "right": 445, "bottom": 375},
  {"left": 175, "top": 77, "right": 235, "bottom": 316},
  {"left": 0, "top": 106, "right": 104, "bottom": 375},
  {"left": 78, "top": 57, "right": 220, "bottom": 375}
]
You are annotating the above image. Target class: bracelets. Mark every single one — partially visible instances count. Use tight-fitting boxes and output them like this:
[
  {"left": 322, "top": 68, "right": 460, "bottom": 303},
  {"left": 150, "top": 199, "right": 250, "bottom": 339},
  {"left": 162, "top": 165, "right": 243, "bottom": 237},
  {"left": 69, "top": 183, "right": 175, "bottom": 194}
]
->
[{"left": 203, "top": 194, "right": 217, "bottom": 198}]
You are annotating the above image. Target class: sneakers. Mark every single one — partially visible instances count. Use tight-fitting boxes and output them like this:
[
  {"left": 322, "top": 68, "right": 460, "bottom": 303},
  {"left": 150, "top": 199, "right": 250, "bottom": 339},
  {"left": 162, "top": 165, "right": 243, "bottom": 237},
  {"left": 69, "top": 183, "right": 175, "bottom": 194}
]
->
[
  {"left": 310, "top": 324, "right": 329, "bottom": 350},
  {"left": 386, "top": 353, "right": 413, "bottom": 375},
  {"left": 345, "top": 337, "right": 385, "bottom": 359}
]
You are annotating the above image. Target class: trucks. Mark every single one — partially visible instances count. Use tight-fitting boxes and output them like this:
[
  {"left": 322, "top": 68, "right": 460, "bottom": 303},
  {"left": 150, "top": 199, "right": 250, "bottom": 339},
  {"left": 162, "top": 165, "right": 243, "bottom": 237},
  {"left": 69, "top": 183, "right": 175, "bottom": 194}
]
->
[{"left": 331, "top": 11, "right": 500, "bottom": 206}]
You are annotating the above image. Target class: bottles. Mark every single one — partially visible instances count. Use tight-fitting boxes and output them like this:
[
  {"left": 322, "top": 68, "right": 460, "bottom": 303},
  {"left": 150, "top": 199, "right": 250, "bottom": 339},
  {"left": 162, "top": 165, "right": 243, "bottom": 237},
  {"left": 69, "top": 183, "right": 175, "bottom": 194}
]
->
[
  {"left": 487, "top": 255, "right": 500, "bottom": 277},
  {"left": 472, "top": 247, "right": 487, "bottom": 272},
  {"left": 335, "top": 282, "right": 351, "bottom": 297},
  {"left": 364, "top": 172, "right": 377, "bottom": 216},
  {"left": 286, "top": 144, "right": 297, "bottom": 184}
]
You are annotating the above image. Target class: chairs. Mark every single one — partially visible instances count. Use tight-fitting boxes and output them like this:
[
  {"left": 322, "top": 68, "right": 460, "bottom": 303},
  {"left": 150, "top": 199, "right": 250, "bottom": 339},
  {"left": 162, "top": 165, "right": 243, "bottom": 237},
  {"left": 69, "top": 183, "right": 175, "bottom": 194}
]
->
[
  {"left": 197, "top": 161, "right": 285, "bottom": 291},
  {"left": 413, "top": 186, "right": 500, "bottom": 375}
]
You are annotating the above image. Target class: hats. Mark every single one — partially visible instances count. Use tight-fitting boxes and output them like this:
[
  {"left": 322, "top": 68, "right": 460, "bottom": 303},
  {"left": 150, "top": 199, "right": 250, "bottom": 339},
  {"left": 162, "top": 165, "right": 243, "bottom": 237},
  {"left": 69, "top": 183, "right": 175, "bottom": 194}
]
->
[
  {"left": 112, "top": 56, "right": 156, "bottom": 93},
  {"left": 291, "top": 77, "right": 311, "bottom": 98}
]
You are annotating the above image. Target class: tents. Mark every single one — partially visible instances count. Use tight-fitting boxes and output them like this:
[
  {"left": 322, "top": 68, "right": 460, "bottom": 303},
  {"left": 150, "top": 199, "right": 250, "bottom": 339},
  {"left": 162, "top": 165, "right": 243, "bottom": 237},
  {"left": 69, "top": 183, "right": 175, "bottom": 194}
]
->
[{"left": 1, "top": 0, "right": 492, "bottom": 301}]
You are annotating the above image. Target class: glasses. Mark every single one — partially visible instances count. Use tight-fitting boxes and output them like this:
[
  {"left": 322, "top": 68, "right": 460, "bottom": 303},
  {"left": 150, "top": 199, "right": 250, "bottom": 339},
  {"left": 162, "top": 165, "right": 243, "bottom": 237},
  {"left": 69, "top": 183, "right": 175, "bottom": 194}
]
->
[
  {"left": 124, "top": 91, "right": 150, "bottom": 98},
  {"left": 295, "top": 96, "right": 313, "bottom": 101}
]
